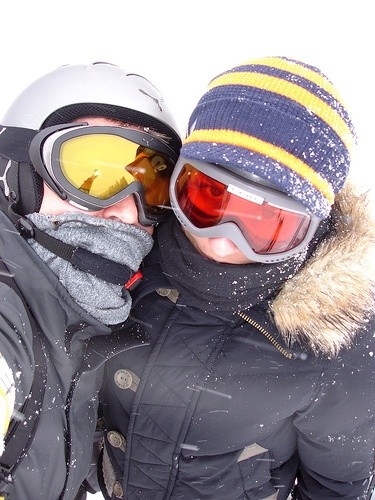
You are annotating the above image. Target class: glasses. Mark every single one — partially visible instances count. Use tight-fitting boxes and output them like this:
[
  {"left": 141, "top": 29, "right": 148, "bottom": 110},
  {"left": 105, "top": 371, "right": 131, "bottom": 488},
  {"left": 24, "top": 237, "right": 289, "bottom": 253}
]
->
[
  {"left": 0, "top": 125, "right": 177, "bottom": 224},
  {"left": 168, "top": 154, "right": 319, "bottom": 264}
]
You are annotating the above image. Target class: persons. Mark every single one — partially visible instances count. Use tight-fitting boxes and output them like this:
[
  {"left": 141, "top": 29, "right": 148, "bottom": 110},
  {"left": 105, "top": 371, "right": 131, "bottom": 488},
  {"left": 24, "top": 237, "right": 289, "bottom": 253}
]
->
[
  {"left": 92, "top": 56, "right": 375, "bottom": 500},
  {"left": 1, "top": 59, "right": 182, "bottom": 500}
]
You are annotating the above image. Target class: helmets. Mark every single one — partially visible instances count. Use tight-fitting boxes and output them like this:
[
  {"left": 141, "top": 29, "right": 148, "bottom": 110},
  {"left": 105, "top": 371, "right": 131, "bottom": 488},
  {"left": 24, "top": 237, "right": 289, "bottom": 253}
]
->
[{"left": 2, "top": 61, "right": 182, "bottom": 235}]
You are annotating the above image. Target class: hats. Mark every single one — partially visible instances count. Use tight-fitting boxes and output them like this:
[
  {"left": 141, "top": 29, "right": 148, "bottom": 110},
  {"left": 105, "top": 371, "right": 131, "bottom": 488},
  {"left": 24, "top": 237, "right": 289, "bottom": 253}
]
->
[{"left": 181, "top": 56, "right": 358, "bottom": 218}]
